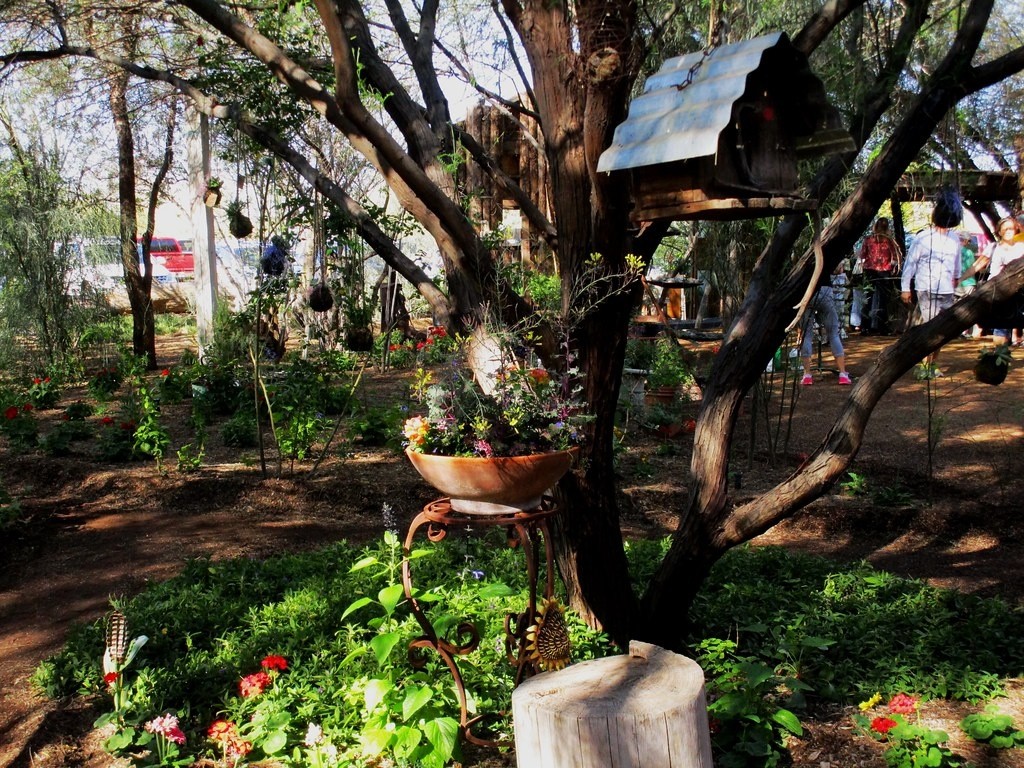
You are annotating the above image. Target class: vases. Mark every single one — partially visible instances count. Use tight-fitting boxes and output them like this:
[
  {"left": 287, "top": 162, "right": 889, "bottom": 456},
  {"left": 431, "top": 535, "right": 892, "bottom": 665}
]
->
[{"left": 403, "top": 447, "right": 578, "bottom": 516}]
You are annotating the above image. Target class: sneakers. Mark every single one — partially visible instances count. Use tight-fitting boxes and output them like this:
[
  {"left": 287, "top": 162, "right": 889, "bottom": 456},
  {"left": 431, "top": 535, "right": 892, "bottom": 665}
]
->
[{"left": 914, "top": 363, "right": 945, "bottom": 380}]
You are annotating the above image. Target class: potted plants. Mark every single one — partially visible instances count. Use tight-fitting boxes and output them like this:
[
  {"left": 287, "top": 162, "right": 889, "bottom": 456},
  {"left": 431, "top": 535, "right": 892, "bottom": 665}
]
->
[{"left": 643, "top": 338, "right": 690, "bottom": 436}]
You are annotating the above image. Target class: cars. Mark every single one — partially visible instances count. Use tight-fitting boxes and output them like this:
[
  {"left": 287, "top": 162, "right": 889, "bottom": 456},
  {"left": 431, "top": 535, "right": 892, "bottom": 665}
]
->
[{"left": 51, "top": 234, "right": 385, "bottom": 298}]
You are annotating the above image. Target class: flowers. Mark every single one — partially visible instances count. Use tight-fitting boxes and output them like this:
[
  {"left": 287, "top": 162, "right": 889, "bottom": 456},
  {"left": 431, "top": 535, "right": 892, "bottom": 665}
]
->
[{"left": 389, "top": 254, "right": 646, "bottom": 457}]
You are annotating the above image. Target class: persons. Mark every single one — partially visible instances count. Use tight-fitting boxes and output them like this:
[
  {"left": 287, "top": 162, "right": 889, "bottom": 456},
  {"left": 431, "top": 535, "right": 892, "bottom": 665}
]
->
[
  {"left": 901, "top": 215, "right": 962, "bottom": 379},
  {"left": 960, "top": 217, "right": 1024, "bottom": 352},
  {"left": 798, "top": 264, "right": 851, "bottom": 385},
  {"left": 955, "top": 231, "right": 976, "bottom": 339},
  {"left": 831, "top": 217, "right": 923, "bottom": 337}
]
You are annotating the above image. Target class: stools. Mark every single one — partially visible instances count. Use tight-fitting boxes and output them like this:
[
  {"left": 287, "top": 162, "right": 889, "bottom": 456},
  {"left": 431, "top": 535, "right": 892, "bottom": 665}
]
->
[{"left": 402, "top": 495, "right": 567, "bottom": 747}]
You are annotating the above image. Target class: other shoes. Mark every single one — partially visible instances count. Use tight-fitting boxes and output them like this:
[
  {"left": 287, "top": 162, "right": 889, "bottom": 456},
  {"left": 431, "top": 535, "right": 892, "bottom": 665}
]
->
[
  {"left": 960, "top": 326, "right": 983, "bottom": 339},
  {"left": 861, "top": 327, "right": 871, "bottom": 336},
  {"left": 801, "top": 374, "right": 812, "bottom": 385},
  {"left": 1011, "top": 340, "right": 1020, "bottom": 346},
  {"left": 840, "top": 328, "right": 848, "bottom": 339},
  {"left": 838, "top": 372, "right": 852, "bottom": 384},
  {"left": 871, "top": 326, "right": 889, "bottom": 336}
]
